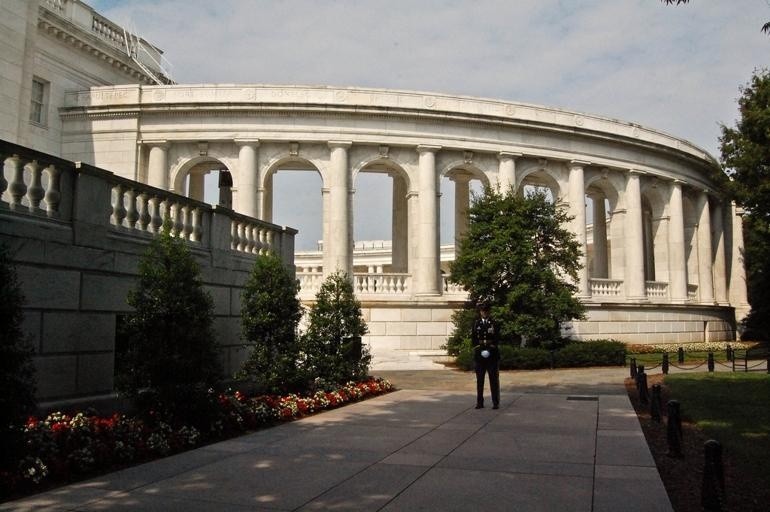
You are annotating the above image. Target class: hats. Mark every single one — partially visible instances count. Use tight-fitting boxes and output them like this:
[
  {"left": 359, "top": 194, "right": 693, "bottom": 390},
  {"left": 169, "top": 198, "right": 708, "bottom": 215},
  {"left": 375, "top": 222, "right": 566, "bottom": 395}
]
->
[{"left": 478, "top": 304, "right": 491, "bottom": 311}]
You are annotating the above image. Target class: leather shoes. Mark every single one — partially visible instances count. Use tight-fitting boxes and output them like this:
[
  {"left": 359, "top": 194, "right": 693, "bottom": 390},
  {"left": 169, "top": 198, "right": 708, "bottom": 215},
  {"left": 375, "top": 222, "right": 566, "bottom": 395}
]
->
[
  {"left": 475, "top": 405, "right": 483, "bottom": 408},
  {"left": 492, "top": 404, "right": 499, "bottom": 409}
]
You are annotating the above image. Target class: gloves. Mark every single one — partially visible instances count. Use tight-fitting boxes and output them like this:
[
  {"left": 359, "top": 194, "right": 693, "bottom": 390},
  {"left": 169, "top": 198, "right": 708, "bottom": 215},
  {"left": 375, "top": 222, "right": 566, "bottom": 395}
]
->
[{"left": 481, "top": 350, "right": 489, "bottom": 357}]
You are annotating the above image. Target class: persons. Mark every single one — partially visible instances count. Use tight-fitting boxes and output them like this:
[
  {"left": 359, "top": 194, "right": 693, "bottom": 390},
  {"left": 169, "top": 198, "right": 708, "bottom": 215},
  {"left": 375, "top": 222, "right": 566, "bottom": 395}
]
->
[{"left": 472, "top": 303, "right": 500, "bottom": 410}]
